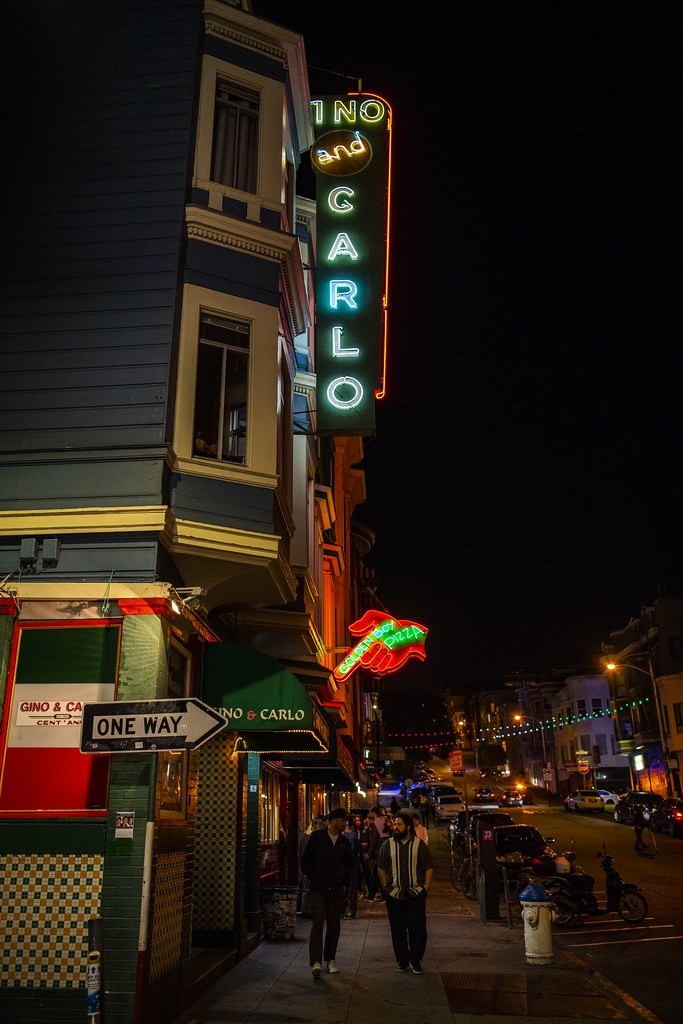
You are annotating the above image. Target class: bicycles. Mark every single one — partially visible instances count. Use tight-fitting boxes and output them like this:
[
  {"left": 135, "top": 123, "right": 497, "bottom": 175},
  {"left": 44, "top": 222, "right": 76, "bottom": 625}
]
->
[{"left": 451, "top": 830, "right": 478, "bottom": 901}]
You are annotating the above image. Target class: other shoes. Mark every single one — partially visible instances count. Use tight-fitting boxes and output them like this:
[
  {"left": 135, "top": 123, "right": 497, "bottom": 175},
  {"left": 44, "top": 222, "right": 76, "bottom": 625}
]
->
[
  {"left": 344, "top": 913, "right": 355, "bottom": 919},
  {"left": 363, "top": 898, "right": 374, "bottom": 902},
  {"left": 396, "top": 962, "right": 409, "bottom": 971},
  {"left": 327, "top": 960, "right": 338, "bottom": 973},
  {"left": 312, "top": 962, "right": 321, "bottom": 976},
  {"left": 409, "top": 960, "right": 423, "bottom": 974}
]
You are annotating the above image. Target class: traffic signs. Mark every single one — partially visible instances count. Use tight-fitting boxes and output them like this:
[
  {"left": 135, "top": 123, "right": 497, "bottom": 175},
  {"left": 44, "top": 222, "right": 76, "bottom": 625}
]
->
[{"left": 80, "top": 697, "right": 231, "bottom": 756}]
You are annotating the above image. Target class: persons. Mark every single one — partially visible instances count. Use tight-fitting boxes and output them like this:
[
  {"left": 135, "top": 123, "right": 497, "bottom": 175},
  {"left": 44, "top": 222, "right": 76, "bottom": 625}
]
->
[
  {"left": 377, "top": 813, "right": 434, "bottom": 973},
  {"left": 632, "top": 809, "right": 647, "bottom": 851},
  {"left": 391, "top": 797, "right": 398, "bottom": 813},
  {"left": 412, "top": 813, "right": 429, "bottom": 846},
  {"left": 420, "top": 794, "right": 430, "bottom": 829},
  {"left": 301, "top": 808, "right": 352, "bottom": 977},
  {"left": 303, "top": 806, "right": 396, "bottom": 920}
]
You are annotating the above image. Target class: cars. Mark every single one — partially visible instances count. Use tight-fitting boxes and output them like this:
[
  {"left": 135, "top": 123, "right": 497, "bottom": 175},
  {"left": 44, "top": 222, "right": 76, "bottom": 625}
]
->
[
  {"left": 605, "top": 798, "right": 620, "bottom": 815},
  {"left": 474, "top": 787, "right": 496, "bottom": 803},
  {"left": 647, "top": 797, "right": 683, "bottom": 836},
  {"left": 453, "top": 769, "right": 464, "bottom": 777},
  {"left": 508, "top": 786, "right": 533, "bottom": 806},
  {"left": 598, "top": 790, "right": 617, "bottom": 805},
  {"left": 614, "top": 790, "right": 665, "bottom": 826},
  {"left": 497, "top": 790, "right": 524, "bottom": 807},
  {"left": 564, "top": 789, "right": 606, "bottom": 813},
  {"left": 407, "top": 769, "right": 558, "bottom": 889},
  {"left": 479, "top": 768, "right": 505, "bottom": 779}
]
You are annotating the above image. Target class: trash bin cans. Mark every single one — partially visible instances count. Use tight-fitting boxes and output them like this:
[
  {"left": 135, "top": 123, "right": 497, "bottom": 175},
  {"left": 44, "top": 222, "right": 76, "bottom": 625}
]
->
[{"left": 262, "top": 885, "right": 300, "bottom": 941}]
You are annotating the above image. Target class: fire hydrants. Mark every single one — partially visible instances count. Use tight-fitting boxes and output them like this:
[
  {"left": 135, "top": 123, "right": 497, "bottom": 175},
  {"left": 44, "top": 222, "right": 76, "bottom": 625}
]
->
[{"left": 518, "top": 883, "right": 557, "bottom": 967}]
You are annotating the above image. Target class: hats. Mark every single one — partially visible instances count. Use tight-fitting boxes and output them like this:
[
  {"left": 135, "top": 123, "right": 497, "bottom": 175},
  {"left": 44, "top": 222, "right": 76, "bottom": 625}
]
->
[{"left": 328, "top": 807, "right": 352, "bottom": 819}]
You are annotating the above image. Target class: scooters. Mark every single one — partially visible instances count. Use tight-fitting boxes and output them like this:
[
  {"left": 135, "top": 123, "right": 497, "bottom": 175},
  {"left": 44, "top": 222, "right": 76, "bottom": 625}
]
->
[{"left": 544, "top": 842, "right": 651, "bottom": 926}]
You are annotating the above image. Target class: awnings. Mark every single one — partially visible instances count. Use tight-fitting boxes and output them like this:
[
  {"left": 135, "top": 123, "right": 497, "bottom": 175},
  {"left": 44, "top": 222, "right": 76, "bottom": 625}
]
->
[{"left": 204, "top": 641, "right": 356, "bottom": 792}]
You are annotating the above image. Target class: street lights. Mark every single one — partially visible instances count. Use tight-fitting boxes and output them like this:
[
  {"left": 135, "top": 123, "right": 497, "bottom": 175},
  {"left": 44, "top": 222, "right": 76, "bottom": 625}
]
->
[
  {"left": 514, "top": 714, "right": 554, "bottom": 808},
  {"left": 607, "top": 657, "right": 675, "bottom": 798}
]
